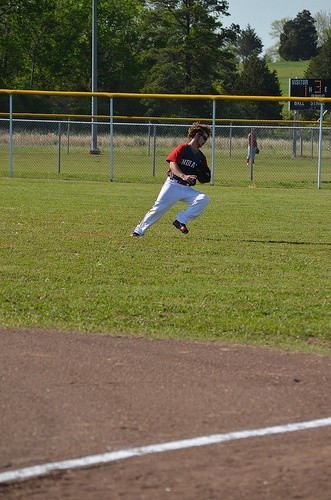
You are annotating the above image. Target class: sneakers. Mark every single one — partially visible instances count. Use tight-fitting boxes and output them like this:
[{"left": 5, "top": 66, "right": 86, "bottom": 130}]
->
[
  {"left": 173, "top": 220, "right": 188, "bottom": 234},
  {"left": 132, "top": 232, "right": 139, "bottom": 237}
]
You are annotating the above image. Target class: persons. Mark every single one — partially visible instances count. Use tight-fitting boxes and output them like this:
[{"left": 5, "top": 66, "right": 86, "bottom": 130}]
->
[
  {"left": 131, "top": 123, "right": 212, "bottom": 237},
  {"left": 245, "top": 128, "right": 260, "bottom": 167}
]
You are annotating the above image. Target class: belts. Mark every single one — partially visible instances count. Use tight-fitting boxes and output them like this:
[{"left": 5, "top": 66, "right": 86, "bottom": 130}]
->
[{"left": 170, "top": 178, "right": 189, "bottom": 186}]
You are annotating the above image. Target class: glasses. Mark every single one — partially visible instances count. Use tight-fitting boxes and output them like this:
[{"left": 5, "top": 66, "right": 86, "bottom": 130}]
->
[{"left": 199, "top": 133, "right": 207, "bottom": 141}]
